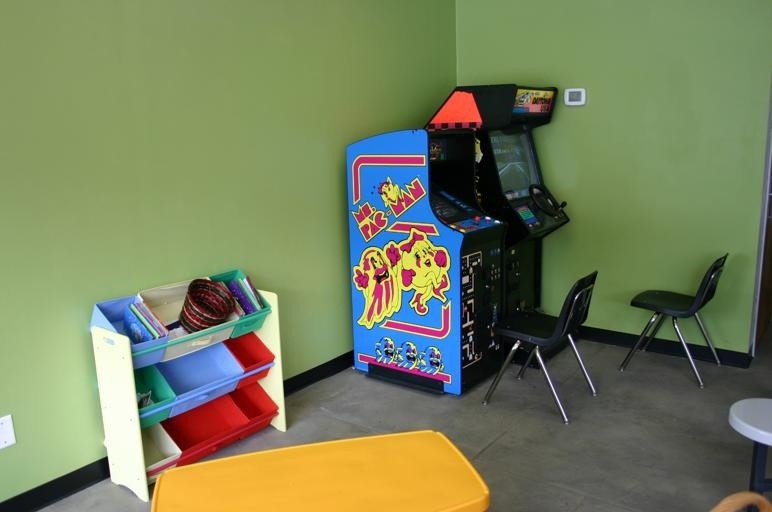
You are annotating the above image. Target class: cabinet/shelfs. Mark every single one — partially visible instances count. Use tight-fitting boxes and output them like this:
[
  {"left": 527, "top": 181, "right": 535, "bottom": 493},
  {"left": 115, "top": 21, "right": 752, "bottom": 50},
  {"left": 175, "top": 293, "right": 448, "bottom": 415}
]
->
[{"left": 89, "top": 289, "right": 287, "bottom": 503}]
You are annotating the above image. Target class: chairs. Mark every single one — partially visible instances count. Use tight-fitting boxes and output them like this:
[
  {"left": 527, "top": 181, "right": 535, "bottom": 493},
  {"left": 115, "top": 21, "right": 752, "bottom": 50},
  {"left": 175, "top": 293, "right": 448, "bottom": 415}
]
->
[
  {"left": 618, "top": 251, "right": 730, "bottom": 389},
  {"left": 482, "top": 270, "right": 599, "bottom": 423}
]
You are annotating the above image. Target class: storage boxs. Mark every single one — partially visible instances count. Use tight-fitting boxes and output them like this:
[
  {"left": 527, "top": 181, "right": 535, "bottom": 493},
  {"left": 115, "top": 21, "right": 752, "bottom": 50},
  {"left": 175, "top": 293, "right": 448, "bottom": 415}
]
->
[
  {"left": 132, "top": 367, "right": 175, "bottom": 428},
  {"left": 229, "top": 380, "right": 278, "bottom": 439},
  {"left": 161, "top": 394, "right": 249, "bottom": 467},
  {"left": 221, "top": 330, "right": 274, "bottom": 392},
  {"left": 89, "top": 295, "right": 166, "bottom": 368},
  {"left": 209, "top": 269, "right": 272, "bottom": 342},
  {"left": 138, "top": 275, "right": 234, "bottom": 361},
  {"left": 152, "top": 342, "right": 243, "bottom": 420},
  {"left": 139, "top": 421, "right": 181, "bottom": 484}
]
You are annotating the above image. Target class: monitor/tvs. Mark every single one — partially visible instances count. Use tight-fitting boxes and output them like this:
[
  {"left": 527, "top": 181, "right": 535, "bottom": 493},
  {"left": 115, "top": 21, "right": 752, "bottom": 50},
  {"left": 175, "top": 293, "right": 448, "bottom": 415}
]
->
[
  {"left": 429, "top": 187, "right": 472, "bottom": 223},
  {"left": 489, "top": 131, "right": 542, "bottom": 200}
]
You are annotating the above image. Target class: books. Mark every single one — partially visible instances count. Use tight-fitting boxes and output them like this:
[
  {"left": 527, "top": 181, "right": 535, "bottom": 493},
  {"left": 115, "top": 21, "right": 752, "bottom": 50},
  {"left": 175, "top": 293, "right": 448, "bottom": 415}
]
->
[
  {"left": 122, "top": 302, "right": 167, "bottom": 345},
  {"left": 216, "top": 275, "right": 264, "bottom": 318}
]
